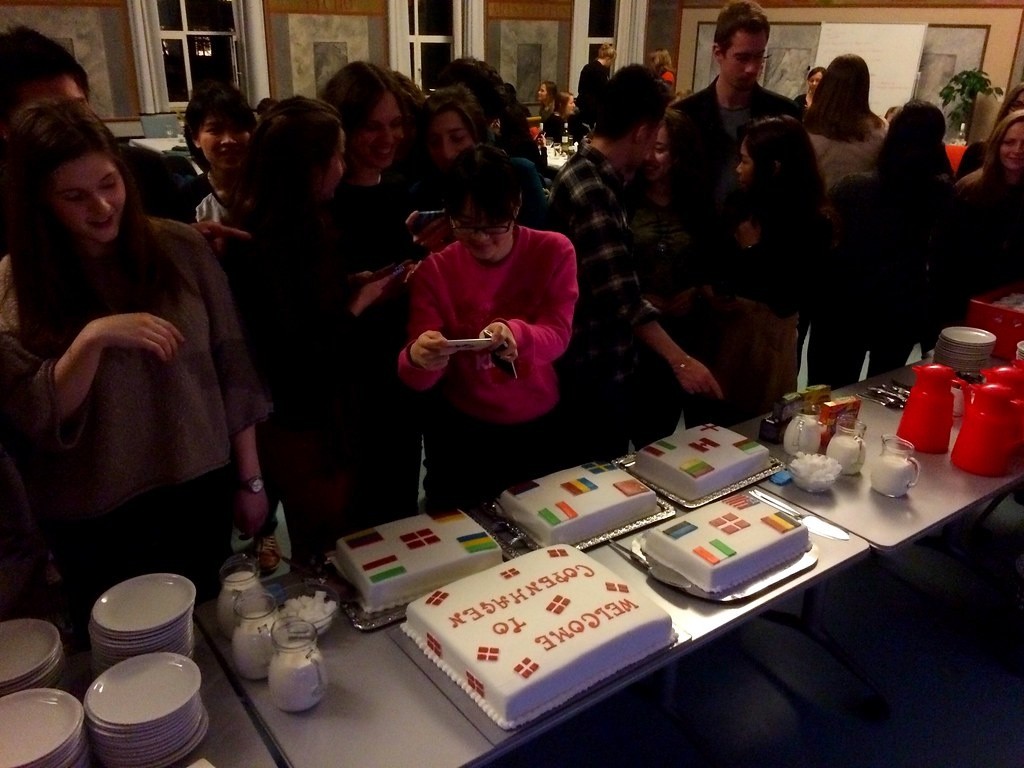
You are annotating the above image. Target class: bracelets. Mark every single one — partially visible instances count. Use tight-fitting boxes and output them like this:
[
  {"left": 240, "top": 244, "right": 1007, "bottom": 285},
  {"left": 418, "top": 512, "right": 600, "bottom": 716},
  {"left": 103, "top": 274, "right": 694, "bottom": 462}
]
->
[{"left": 675, "top": 356, "right": 690, "bottom": 372}]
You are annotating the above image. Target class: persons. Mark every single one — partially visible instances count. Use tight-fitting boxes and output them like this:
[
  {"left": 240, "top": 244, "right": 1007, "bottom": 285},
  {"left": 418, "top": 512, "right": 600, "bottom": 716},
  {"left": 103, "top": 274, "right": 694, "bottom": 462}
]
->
[
  {"left": 0, "top": 0, "right": 1024, "bottom": 658},
  {"left": 505, "top": 82, "right": 531, "bottom": 118},
  {"left": 538, "top": 81, "right": 556, "bottom": 120},
  {"left": 650, "top": 49, "right": 675, "bottom": 89},
  {"left": 544, "top": 92, "right": 575, "bottom": 144},
  {"left": 576, "top": 45, "right": 617, "bottom": 108},
  {"left": 793, "top": 68, "right": 826, "bottom": 118}
]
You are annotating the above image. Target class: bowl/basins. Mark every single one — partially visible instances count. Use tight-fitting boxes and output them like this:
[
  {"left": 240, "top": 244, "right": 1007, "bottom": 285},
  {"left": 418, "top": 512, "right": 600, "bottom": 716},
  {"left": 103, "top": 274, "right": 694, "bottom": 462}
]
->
[
  {"left": 786, "top": 451, "right": 843, "bottom": 493},
  {"left": 273, "top": 582, "right": 341, "bottom": 636}
]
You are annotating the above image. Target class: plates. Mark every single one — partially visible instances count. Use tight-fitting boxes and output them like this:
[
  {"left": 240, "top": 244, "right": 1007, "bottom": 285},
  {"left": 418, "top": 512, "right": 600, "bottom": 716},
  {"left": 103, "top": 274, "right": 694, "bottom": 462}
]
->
[
  {"left": 88, "top": 573, "right": 197, "bottom": 679},
  {"left": 1016, "top": 341, "right": 1024, "bottom": 360},
  {"left": 0, "top": 688, "right": 93, "bottom": 768},
  {"left": 0, "top": 619, "right": 76, "bottom": 698},
  {"left": 933, "top": 327, "right": 997, "bottom": 375},
  {"left": 83, "top": 652, "right": 210, "bottom": 768}
]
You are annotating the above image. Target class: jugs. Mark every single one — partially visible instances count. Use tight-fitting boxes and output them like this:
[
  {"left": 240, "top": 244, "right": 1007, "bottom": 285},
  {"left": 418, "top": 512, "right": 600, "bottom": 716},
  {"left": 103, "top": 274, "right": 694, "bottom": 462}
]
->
[{"left": 896, "top": 360, "right": 1024, "bottom": 477}]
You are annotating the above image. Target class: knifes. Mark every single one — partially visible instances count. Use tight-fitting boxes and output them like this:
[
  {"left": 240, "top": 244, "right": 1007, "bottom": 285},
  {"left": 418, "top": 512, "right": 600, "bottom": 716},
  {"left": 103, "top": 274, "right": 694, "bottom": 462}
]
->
[{"left": 749, "top": 488, "right": 850, "bottom": 541}]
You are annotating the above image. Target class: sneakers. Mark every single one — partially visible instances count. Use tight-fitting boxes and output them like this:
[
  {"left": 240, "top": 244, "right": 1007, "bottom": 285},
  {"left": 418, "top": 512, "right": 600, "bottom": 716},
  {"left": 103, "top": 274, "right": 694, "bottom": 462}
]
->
[{"left": 251, "top": 534, "right": 283, "bottom": 573}]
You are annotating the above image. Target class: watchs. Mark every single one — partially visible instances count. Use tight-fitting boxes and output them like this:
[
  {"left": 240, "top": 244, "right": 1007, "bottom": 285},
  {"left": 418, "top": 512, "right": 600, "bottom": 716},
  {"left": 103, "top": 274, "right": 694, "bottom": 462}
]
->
[{"left": 241, "top": 476, "right": 264, "bottom": 493}]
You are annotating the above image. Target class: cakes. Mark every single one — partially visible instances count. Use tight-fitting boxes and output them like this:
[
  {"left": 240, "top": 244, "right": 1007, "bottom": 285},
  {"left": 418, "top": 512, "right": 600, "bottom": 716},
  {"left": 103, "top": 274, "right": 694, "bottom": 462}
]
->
[
  {"left": 634, "top": 423, "right": 770, "bottom": 502},
  {"left": 332, "top": 505, "right": 501, "bottom": 614},
  {"left": 400, "top": 542, "right": 679, "bottom": 728},
  {"left": 496, "top": 459, "right": 657, "bottom": 550},
  {"left": 642, "top": 492, "right": 812, "bottom": 593}
]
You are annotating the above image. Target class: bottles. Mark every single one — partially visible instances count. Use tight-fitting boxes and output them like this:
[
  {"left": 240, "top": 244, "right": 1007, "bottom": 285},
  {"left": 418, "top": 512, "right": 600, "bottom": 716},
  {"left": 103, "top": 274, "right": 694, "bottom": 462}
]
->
[
  {"left": 956, "top": 123, "right": 966, "bottom": 145},
  {"left": 561, "top": 123, "right": 569, "bottom": 147}
]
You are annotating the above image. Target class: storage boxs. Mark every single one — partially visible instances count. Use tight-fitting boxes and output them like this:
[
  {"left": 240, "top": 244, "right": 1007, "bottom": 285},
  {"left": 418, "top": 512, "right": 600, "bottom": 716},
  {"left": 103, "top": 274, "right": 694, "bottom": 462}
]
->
[{"left": 966, "top": 280, "right": 1024, "bottom": 360}]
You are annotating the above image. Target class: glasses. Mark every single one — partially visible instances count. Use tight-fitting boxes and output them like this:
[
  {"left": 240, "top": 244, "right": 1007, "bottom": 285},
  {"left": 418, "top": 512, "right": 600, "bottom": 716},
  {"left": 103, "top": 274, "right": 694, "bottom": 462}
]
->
[
  {"left": 1009, "top": 101, "right": 1024, "bottom": 112},
  {"left": 448, "top": 213, "right": 514, "bottom": 236}
]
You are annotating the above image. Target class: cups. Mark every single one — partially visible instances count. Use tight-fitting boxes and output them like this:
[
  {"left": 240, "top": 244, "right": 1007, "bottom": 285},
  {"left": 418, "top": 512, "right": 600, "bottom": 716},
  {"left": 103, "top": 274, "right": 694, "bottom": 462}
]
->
[
  {"left": 554, "top": 143, "right": 561, "bottom": 156},
  {"left": 826, "top": 417, "right": 867, "bottom": 474},
  {"left": 783, "top": 401, "right": 821, "bottom": 458},
  {"left": 569, "top": 135, "right": 574, "bottom": 146},
  {"left": 870, "top": 433, "right": 921, "bottom": 498},
  {"left": 215, "top": 552, "right": 330, "bottom": 713},
  {"left": 546, "top": 137, "right": 554, "bottom": 147}
]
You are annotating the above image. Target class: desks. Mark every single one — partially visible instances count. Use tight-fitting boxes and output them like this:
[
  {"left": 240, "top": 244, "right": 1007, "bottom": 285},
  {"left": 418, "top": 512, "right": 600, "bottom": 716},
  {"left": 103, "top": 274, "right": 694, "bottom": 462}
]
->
[{"left": 64, "top": 357, "right": 1024, "bottom": 768}]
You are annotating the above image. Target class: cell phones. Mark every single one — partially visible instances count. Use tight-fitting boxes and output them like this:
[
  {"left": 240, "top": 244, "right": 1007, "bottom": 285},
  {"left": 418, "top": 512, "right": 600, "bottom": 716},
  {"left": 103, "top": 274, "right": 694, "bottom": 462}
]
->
[
  {"left": 484, "top": 330, "right": 517, "bottom": 379},
  {"left": 447, "top": 338, "right": 490, "bottom": 351},
  {"left": 414, "top": 210, "right": 446, "bottom": 235}
]
